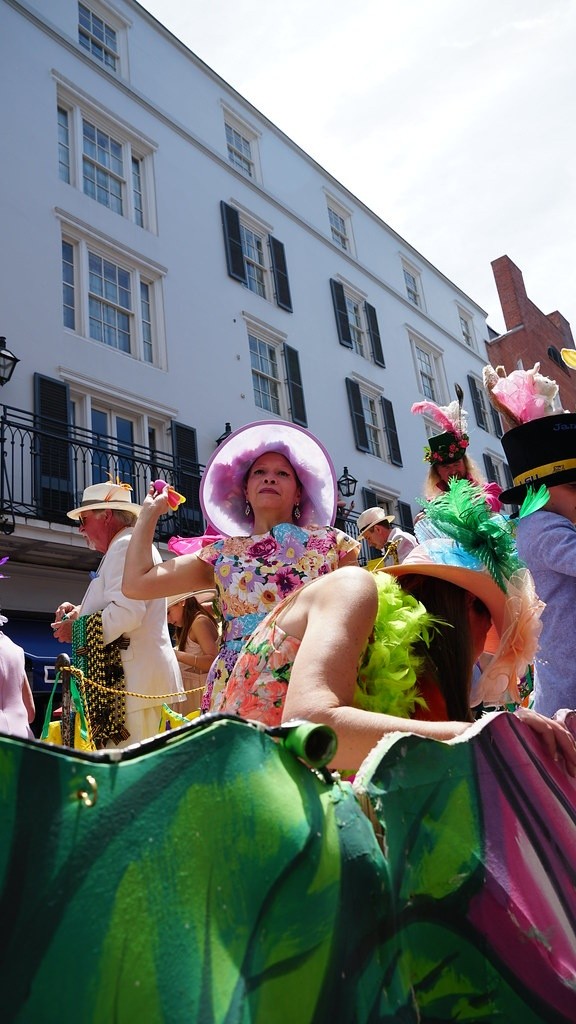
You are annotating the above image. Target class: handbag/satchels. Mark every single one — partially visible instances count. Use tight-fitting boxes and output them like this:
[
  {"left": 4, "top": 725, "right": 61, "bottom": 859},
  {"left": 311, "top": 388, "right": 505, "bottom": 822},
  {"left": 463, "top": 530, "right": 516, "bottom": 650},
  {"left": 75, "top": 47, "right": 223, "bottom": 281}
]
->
[{"left": 39, "top": 670, "right": 95, "bottom": 752}]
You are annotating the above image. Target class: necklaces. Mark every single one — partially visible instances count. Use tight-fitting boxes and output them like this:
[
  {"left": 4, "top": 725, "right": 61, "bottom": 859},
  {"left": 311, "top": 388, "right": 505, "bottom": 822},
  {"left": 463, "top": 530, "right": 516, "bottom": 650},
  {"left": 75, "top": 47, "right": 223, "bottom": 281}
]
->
[{"left": 56, "top": 612, "right": 126, "bottom": 742}]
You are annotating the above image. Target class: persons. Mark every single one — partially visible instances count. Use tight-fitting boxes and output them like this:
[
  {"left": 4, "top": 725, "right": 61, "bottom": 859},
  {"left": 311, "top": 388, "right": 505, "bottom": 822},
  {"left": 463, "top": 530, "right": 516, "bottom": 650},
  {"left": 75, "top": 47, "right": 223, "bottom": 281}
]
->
[
  {"left": 51, "top": 482, "right": 186, "bottom": 749},
  {"left": 0, "top": 615, "right": 35, "bottom": 739},
  {"left": 167, "top": 589, "right": 221, "bottom": 716},
  {"left": 411, "top": 384, "right": 480, "bottom": 525},
  {"left": 356, "top": 507, "right": 419, "bottom": 567},
  {"left": 219, "top": 479, "right": 576, "bottom": 777},
  {"left": 483, "top": 364, "right": 576, "bottom": 717},
  {"left": 121, "top": 419, "right": 362, "bottom": 717}
]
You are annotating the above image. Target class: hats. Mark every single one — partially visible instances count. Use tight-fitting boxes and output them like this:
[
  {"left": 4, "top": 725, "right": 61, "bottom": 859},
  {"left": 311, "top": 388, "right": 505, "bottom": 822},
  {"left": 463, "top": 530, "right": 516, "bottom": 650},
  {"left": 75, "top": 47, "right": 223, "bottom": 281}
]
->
[
  {"left": 372, "top": 476, "right": 550, "bottom": 709},
  {"left": 480, "top": 360, "right": 576, "bottom": 505},
  {"left": 165, "top": 589, "right": 218, "bottom": 608},
  {"left": 66, "top": 482, "right": 142, "bottom": 520},
  {"left": 411, "top": 401, "right": 466, "bottom": 465},
  {"left": 355, "top": 507, "right": 395, "bottom": 541},
  {"left": 199, "top": 420, "right": 338, "bottom": 537}
]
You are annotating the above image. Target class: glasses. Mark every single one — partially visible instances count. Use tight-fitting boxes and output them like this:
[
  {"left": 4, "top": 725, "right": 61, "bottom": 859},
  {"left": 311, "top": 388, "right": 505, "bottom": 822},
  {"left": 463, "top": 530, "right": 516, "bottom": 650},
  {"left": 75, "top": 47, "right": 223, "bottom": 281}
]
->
[{"left": 78, "top": 513, "right": 96, "bottom": 526}]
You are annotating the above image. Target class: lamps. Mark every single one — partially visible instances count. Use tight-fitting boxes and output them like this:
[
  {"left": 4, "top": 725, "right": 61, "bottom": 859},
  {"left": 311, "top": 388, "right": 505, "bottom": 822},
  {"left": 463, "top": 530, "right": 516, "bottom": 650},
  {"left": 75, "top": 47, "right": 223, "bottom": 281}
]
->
[
  {"left": 337, "top": 466, "right": 358, "bottom": 497},
  {"left": 214, "top": 422, "right": 233, "bottom": 446},
  {"left": 0, "top": 336, "right": 21, "bottom": 387}
]
